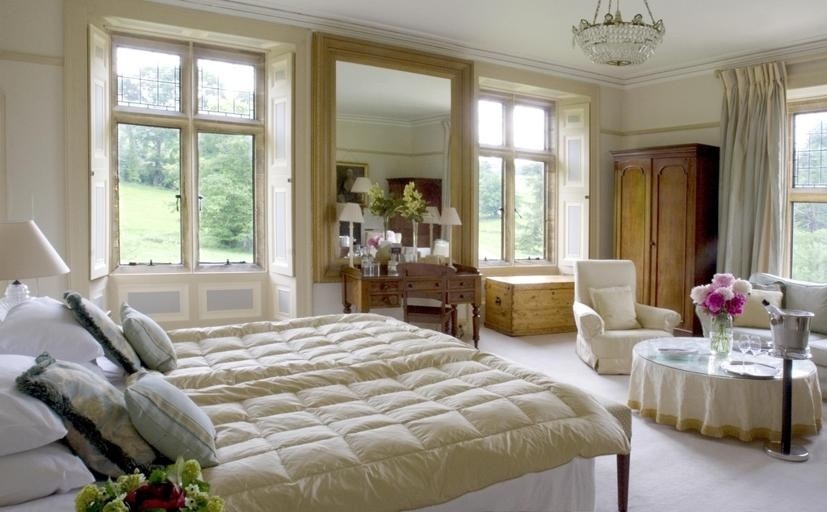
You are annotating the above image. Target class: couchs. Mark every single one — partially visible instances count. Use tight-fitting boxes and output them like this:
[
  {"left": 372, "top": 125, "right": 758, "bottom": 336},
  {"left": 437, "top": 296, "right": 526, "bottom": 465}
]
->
[
  {"left": 572, "top": 258, "right": 681, "bottom": 376},
  {"left": 695, "top": 271, "right": 827, "bottom": 402}
]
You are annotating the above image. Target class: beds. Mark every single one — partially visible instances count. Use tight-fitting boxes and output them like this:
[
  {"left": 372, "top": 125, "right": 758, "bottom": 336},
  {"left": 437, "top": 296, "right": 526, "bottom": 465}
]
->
[{"left": 1, "top": 295, "right": 631, "bottom": 512}]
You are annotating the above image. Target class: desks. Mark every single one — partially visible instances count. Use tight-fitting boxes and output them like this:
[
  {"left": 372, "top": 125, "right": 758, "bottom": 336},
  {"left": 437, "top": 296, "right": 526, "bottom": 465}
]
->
[{"left": 343, "top": 261, "right": 484, "bottom": 352}]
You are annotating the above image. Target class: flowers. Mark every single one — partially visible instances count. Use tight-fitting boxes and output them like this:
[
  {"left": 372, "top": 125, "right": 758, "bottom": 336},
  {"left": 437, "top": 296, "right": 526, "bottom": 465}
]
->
[
  {"left": 367, "top": 184, "right": 397, "bottom": 216},
  {"left": 396, "top": 181, "right": 430, "bottom": 224},
  {"left": 689, "top": 273, "right": 751, "bottom": 318}
]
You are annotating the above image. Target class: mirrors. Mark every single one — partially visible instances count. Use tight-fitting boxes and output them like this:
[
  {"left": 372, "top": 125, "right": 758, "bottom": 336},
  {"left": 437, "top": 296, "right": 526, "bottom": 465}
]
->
[{"left": 312, "top": 30, "right": 496, "bottom": 284}]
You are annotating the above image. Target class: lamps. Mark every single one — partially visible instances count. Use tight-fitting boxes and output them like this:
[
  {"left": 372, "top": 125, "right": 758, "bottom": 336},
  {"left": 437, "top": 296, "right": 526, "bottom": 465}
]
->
[
  {"left": 570, "top": 12, "right": 665, "bottom": 67},
  {"left": 337, "top": 201, "right": 365, "bottom": 270},
  {"left": 437, "top": 207, "right": 463, "bottom": 268},
  {"left": 1, "top": 217, "right": 72, "bottom": 303},
  {"left": 420, "top": 205, "right": 442, "bottom": 255},
  {"left": 339, "top": 201, "right": 365, "bottom": 227},
  {"left": 441, "top": 206, "right": 463, "bottom": 230}
]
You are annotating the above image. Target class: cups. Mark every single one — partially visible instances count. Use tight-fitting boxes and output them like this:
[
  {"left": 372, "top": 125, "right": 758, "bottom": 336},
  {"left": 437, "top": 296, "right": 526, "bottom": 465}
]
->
[
  {"left": 738, "top": 334, "right": 751, "bottom": 370},
  {"left": 751, "top": 337, "right": 762, "bottom": 370}
]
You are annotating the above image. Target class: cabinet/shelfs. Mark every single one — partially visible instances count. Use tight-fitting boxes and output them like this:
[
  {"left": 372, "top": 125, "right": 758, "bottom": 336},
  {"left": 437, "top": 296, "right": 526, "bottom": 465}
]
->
[{"left": 609, "top": 142, "right": 721, "bottom": 338}]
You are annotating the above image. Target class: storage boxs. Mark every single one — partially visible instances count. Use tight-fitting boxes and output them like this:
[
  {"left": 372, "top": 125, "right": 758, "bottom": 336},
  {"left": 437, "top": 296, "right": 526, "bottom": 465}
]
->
[{"left": 487, "top": 274, "right": 583, "bottom": 337}]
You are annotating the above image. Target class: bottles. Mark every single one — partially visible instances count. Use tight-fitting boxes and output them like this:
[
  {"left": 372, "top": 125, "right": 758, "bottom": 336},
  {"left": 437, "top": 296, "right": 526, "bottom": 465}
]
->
[
  {"left": 761, "top": 298, "right": 784, "bottom": 314},
  {"left": 361, "top": 247, "right": 369, "bottom": 273},
  {"left": 355, "top": 244, "right": 362, "bottom": 256}
]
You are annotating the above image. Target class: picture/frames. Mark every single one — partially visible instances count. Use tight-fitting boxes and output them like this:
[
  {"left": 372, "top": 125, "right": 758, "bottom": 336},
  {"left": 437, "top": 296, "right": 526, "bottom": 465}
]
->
[{"left": 333, "top": 161, "right": 370, "bottom": 208}]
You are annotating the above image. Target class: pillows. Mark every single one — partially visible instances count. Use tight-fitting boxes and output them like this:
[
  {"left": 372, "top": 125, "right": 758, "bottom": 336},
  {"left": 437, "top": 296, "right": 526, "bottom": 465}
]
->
[
  {"left": 1, "top": 298, "right": 103, "bottom": 361},
  {"left": 119, "top": 300, "right": 178, "bottom": 373},
  {"left": 1, "top": 444, "right": 95, "bottom": 507},
  {"left": 124, "top": 369, "right": 221, "bottom": 469},
  {"left": 589, "top": 286, "right": 637, "bottom": 332},
  {"left": 64, "top": 292, "right": 143, "bottom": 374},
  {"left": 1, "top": 352, "right": 68, "bottom": 455},
  {"left": 15, "top": 353, "right": 156, "bottom": 476},
  {"left": 734, "top": 280, "right": 782, "bottom": 327}
]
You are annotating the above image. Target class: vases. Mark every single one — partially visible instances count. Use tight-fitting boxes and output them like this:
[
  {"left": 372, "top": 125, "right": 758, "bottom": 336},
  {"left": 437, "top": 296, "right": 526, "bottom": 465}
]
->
[
  {"left": 382, "top": 216, "right": 390, "bottom": 245},
  {"left": 412, "top": 227, "right": 421, "bottom": 266}
]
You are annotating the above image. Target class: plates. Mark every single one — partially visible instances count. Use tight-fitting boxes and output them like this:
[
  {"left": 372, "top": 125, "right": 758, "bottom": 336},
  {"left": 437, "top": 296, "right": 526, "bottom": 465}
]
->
[{"left": 727, "top": 360, "right": 779, "bottom": 377}]
